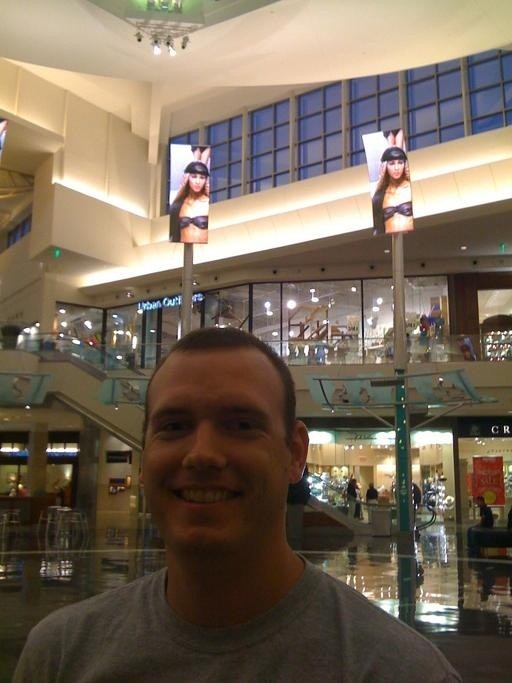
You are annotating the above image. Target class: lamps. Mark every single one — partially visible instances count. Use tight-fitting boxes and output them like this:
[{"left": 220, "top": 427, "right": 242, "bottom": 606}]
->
[{"left": 136, "top": 22, "right": 197, "bottom": 59}]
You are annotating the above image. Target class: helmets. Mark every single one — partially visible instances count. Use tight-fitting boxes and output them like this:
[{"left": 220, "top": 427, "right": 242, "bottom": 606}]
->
[
  {"left": 185, "top": 162, "right": 208, "bottom": 176},
  {"left": 381, "top": 148, "right": 406, "bottom": 161}
]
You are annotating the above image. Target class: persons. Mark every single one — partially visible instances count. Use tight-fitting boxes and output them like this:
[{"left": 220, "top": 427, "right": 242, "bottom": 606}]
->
[
  {"left": 191, "top": 144, "right": 211, "bottom": 165},
  {"left": 11, "top": 326, "right": 461, "bottom": 683},
  {"left": 468, "top": 552, "right": 496, "bottom": 601},
  {"left": 170, "top": 162, "right": 209, "bottom": 243},
  {"left": 467, "top": 496, "right": 493, "bottom": 552},
  {"left": 315, "top": 339, "right": 329, "bottom": 366},
  {"left": 347, "top": 479, "right": 378, "bottom": 525},
  {"left": 383, "top": 128, "right": 403, "bottom": 151},
  {"left": 372, "top": 147, "right": 413, "bottom": 236},
  {"left": 348, "top": 547, "right": 358, "bottom": 572}
]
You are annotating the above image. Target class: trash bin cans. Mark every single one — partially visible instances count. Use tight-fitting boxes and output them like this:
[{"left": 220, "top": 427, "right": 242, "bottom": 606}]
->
[{"left": 372, "top": 509, "right": 391, "bottom": 536}]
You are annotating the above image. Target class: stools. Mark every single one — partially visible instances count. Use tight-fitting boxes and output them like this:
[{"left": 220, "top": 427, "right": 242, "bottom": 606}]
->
[{"left": 467, "top": 525, "right": 512, "bottom": 558}]
